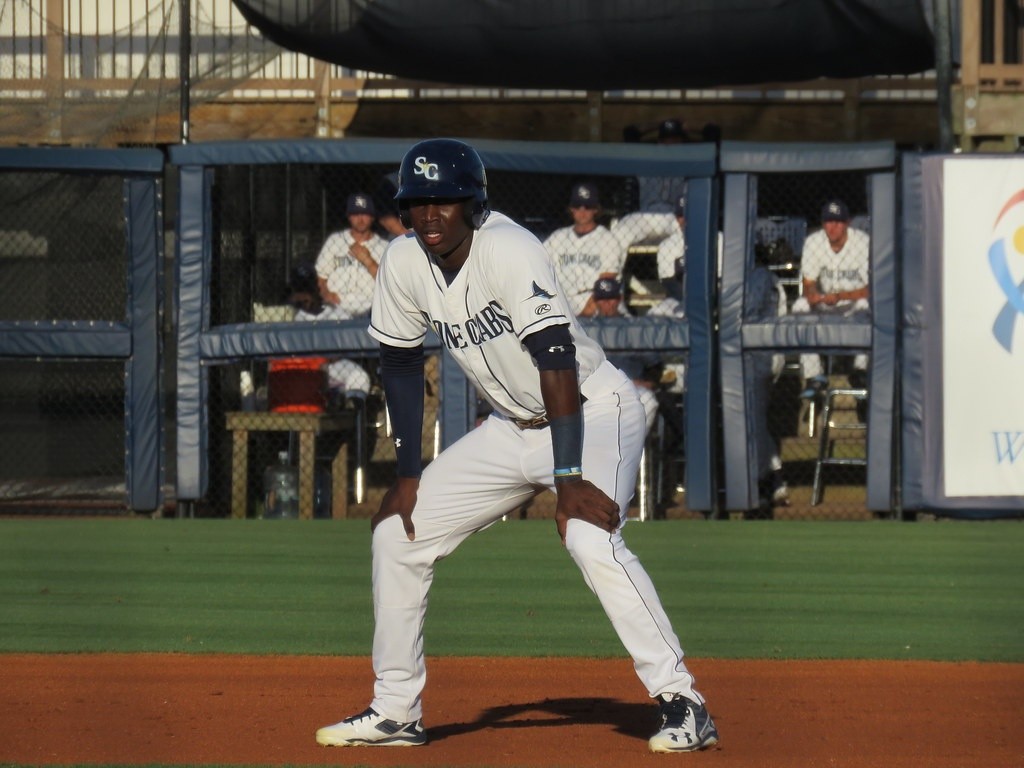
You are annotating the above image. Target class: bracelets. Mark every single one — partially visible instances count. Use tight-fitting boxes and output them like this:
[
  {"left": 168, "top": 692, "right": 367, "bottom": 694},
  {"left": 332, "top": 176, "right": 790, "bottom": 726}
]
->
[{"left": 553, "top": 467, "right": 582, "bottom": 476}]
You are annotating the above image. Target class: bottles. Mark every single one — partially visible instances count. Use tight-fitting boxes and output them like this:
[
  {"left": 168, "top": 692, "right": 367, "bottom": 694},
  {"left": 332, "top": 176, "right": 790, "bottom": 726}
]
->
[{"left": 274, "top": 450, "right": 299, "bottom": 520}]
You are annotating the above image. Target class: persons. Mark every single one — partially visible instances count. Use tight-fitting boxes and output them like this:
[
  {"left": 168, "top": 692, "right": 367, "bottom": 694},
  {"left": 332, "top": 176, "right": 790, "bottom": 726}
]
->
[
  {"left": 240, "top": 279, "right": 370, "bottom": 518},
  {"left": 659, "top": 193, "right": 723, "bottom": 278},
  {"left": 317, "top": 142, "right": 720, "bottom": 753},
  {"left": 380, "top": 215, "right": 440, "bottom": 472},
  {"left": 543, "top": 184, "right": 622, "bottom": 316},
  {"left": 314, "top": 192, "right": 389, "bottom": 317},
  {"left": 750, "top": 232, "right": 792, "bottom": 507},
  {"left": 792, "top": 199, "right": 869, "bottom": 388},
  {"left": 648, "top": 254, "right": 686, "bottom": 504},
  {"left": 590, "top": 280, "right": 660, "bottom": 436}
]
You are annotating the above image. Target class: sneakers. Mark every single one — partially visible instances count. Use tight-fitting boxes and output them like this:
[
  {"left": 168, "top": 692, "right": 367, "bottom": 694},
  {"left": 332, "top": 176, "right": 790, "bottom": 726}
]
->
[
  {"left": 648, "top": 691, "right": 720, "bottom": 753},
  {"left": 315, "top": 707, "right": 428, "bottom": 746}
]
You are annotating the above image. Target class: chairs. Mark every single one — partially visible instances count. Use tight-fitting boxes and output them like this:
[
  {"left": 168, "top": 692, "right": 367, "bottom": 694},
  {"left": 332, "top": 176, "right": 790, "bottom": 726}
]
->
[
  {"left": 225, "top": 353, "right": 355, "bottom": 519},
  {"left": 436, "top": 201, "right": 872, "bottom": 524}
]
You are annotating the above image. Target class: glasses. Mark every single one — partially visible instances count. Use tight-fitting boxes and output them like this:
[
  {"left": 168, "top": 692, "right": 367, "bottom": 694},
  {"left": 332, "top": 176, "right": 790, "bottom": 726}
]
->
[{"left": 571, "top": 204, "right": 594, "bottom": 210}]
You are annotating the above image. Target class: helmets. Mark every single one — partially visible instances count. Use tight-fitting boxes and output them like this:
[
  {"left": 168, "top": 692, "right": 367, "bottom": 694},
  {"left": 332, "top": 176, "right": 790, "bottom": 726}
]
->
[
  {"left": 592, "top": 278, "right": 621, "bottom": 301},
  {"left": 567, "top": 181, "right": 600, "bottom": 208},
  {"left": 393, "top": 138, "right": 491, "bottom": 232},
  {"left": 347, "top": 188, "right": 375, "bottom": 216},
  {"left": 819, "top": 192, "right": 849, "bottom": 222}
]
